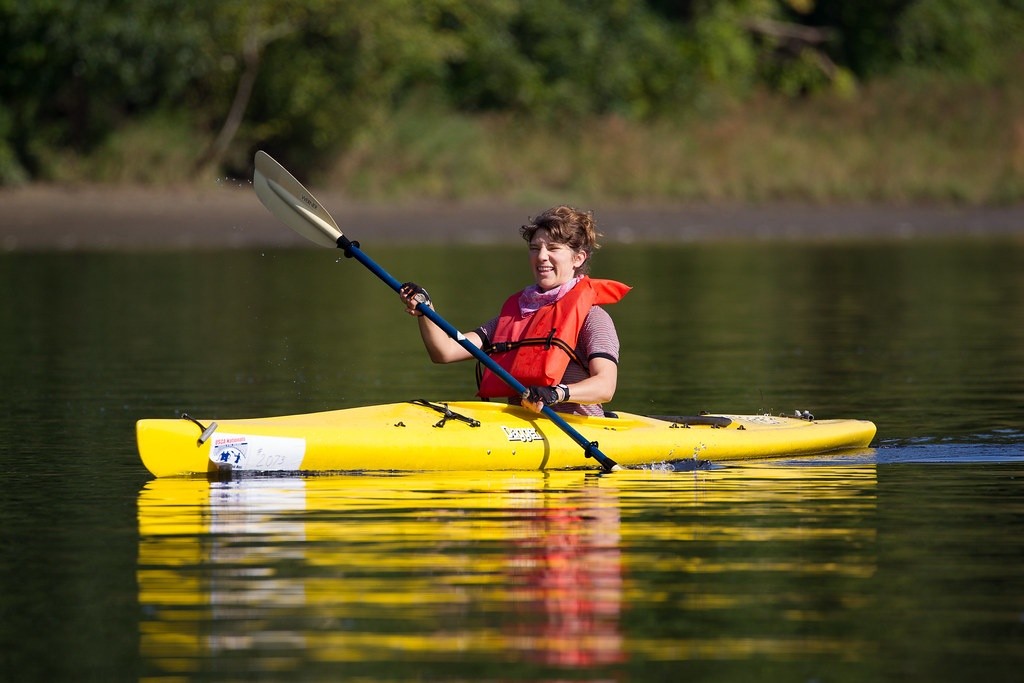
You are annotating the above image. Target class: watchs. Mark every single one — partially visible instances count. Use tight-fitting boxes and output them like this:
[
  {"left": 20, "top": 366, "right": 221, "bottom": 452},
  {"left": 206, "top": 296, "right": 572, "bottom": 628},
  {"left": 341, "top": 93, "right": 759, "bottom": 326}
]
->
[{"left": 557, "top": 383, "right": 570, "bottom": 402}]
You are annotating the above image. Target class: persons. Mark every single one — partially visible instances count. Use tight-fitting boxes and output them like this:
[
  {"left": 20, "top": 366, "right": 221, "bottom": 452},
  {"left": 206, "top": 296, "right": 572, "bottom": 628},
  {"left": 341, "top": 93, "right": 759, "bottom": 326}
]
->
[{"left": 399, "top": 208, "right": 619, "bottom": 417}]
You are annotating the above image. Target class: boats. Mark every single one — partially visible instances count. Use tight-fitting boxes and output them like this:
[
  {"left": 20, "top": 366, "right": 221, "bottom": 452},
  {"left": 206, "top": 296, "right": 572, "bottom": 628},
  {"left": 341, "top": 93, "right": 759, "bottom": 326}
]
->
[
  {"left": 137, "top": 447, "right": 878, "bottom": 538},
  {"left": 135, "top": 401, "right": 876, "bottom": 478}
]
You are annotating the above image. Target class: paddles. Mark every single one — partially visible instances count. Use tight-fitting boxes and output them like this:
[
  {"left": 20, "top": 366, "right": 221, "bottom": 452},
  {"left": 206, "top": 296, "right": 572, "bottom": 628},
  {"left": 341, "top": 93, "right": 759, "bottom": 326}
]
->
[{"left": 249, "top": 146, "right": 626, "bottom": 470}]
